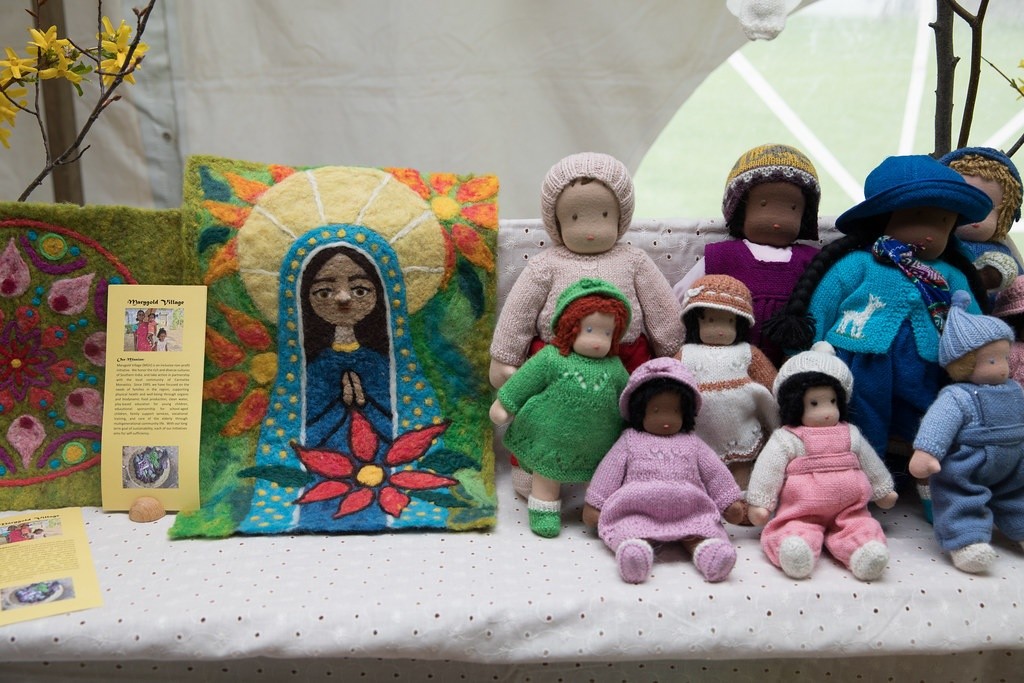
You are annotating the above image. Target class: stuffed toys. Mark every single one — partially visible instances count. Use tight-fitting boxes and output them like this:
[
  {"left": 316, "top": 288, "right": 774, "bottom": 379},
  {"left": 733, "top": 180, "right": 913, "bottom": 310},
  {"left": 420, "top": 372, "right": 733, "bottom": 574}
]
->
[
  {"left": 583, "top": 355, "right": 743, "bottom": 583},
  {"left": 909, "top": 291, "right": 1024, "bottom": 573},
  {"left": 675, "top": 273, "right": 779, "bottom": 526},
  {"left": 769, "top": 155, "right": 987, "bottom": 522},
  {"left": 670, "top": 142, "right": 823, "bottom": 367},
  {"left": 489, "top": 278, "right": 633, "bottom": 538},
  {"left": 745, "top": 340, "right": 899, "bottom": 578},
  {"left": 490, "top": 150, "right": 685, "bottom": 498},
  {"left": 937, "top": 147, "right": 1024, "bottom": 383}
]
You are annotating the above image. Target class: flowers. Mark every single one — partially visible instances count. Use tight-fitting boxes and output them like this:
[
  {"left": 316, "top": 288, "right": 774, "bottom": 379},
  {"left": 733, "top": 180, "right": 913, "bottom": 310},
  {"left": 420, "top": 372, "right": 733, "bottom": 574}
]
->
[{"left": 0, "top": 0, "right": 155, "bottom": 203}]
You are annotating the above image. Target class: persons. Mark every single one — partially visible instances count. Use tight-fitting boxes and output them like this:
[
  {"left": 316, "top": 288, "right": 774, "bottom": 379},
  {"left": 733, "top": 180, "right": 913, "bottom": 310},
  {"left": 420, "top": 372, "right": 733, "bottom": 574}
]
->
[
  {"left": 133, "top": 309, "right": 167, "bottom": 351},
  {"left": 6, "top": 523, "right": 46, "bottom": 543}
]
